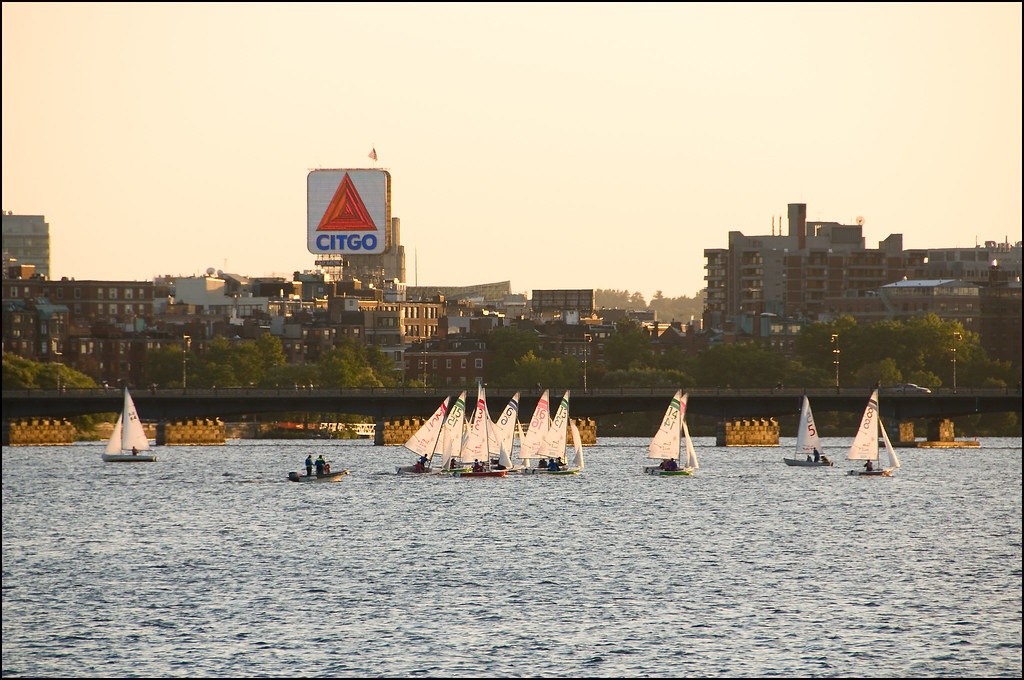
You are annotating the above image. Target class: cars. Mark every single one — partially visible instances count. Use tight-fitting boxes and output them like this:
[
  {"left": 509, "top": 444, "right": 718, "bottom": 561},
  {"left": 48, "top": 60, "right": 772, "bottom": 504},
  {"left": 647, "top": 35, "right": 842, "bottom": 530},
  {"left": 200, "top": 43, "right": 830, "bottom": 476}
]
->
[{"left": 896, "top": 383, "right": 931, "bottom": 393}]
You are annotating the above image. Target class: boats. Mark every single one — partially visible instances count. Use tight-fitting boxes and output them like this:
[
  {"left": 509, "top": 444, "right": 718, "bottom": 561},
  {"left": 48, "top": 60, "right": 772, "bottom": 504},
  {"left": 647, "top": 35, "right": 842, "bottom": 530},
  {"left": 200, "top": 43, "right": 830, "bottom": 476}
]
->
[{"left": 285, "top": 469, "right": 351, "bottom": 482}]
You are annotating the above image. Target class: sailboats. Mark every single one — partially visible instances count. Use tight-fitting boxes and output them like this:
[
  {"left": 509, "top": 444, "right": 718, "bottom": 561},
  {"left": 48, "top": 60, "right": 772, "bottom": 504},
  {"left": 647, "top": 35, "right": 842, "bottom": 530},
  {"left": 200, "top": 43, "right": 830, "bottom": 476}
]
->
[
  {"left": 102, "top": 388, "right": 159, "bottom": 462},
  {"left": 484, "top": 391, "right": 531, "bottom": 474},
  {"left": 516, "top": 388, "right": 584, "bottom": 475},
  {"left": 844, "top": 388, "right": 900, "bottom": 476},
  {"left": 394, "top": 395, "right": 450, "bottom": 475},
  {"left": 641, "top": 389, "right": 700, "bottom": 476},
  {"left": 453, "top": 381, "right": 512, "bottom": 477},
  {"left": 783, "top": 394, "right": 834, "bottom": 467},
  {"left": 431, "top": 391, "right": 473, "bottom": 474}
]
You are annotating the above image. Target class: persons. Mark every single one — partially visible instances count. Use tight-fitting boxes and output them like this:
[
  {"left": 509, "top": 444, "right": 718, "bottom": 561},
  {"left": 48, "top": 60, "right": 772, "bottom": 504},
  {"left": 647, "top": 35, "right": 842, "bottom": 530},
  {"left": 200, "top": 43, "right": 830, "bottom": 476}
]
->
[
  {"left": 413, "top": 461, "right": 425, "bottom": 473},
  {"left": 806, "top": 455, "right": 812, "bottom": 462},
  {"left": 538, "top": 456, "right": 567, "bottom": 472},
  {"left": 821, "top": 456, "right": 828, "bottom": 463},
  {"left": 131, "top": 446, "right": 138, "bottom": 456},
  {"left": 813, "top": 447, "right": 820, "bottom": 462},
  {"left": 864, "top": 459, "right": 873, "bottom": 471},
  {"left": 659, "top": 457, "right": 679, "bottom": 471},
  {"left": 451, "top": 458, "right": 457, "bottom": 469},
  {"left": 419, "top": 453, "right": 430, "bottom": 467},
  {"left": 472, "top": 459, "right": 486, "bottom": 472},
  {"left": 305, "top": 455, "right": 314, "bottom": 476},
  {"left": 314, "top": 455, "right": 326, "bottom": 475}
]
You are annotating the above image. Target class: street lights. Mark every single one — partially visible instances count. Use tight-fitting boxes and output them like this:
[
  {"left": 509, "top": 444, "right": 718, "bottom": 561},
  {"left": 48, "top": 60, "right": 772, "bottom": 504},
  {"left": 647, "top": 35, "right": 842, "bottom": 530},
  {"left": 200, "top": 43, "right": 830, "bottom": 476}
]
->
[
  {"left": 417, "top": 336, "right": 426, "bottom": 393},
  {"left": 830, "top": 334, "right": 840, "bottom": 394},
  {"left": 953, "top": 332, "right": 962, "bottom": 393},
  {"left": 583, "top": 334, "right": 592, "bottom": 393},
  {"left": 182, "top": 335, "right": 190, "bottom": 388}
]
